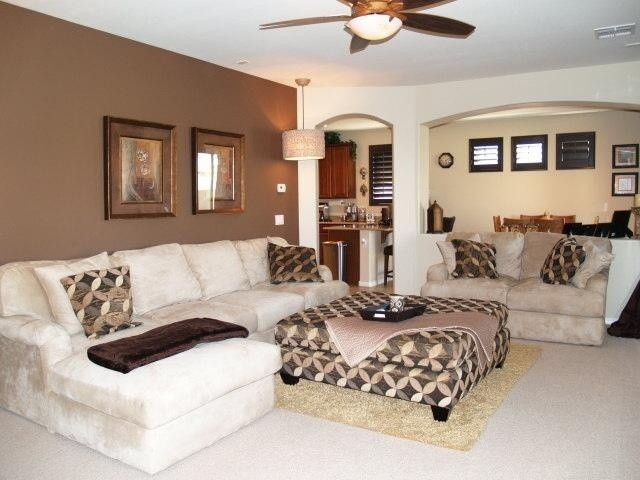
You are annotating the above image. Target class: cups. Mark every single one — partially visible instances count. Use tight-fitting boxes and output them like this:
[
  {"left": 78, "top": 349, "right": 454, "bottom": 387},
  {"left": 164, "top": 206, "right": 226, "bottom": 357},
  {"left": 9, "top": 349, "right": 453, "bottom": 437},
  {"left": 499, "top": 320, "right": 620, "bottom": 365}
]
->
[{"left": 389, "top": 295, "right": 404, "bottom": 313}]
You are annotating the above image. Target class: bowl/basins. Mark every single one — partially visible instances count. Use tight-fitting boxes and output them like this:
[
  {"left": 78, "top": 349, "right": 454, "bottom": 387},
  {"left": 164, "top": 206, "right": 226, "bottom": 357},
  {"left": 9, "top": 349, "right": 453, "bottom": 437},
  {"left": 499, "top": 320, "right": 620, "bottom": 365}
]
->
[{"left": 442, "top": 217, "right": 455, "bottom": 232}]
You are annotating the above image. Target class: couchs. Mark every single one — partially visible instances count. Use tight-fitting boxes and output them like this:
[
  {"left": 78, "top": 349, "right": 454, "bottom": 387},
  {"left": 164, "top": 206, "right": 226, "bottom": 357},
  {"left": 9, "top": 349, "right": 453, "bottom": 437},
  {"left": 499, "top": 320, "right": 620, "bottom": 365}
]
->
[
  {"left": 0, "top": 235, "right": 353, "bottom": 476},
  {"left": 419, "top": 226, "right": 620, "bottom": 351}
]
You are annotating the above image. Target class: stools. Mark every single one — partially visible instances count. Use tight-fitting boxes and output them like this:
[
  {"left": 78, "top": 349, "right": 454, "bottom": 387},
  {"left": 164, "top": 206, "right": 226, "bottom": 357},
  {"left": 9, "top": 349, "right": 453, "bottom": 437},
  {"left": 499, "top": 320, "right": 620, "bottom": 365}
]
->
[{"left": 382, "top": 244, "right": 394, "bottom": 286}]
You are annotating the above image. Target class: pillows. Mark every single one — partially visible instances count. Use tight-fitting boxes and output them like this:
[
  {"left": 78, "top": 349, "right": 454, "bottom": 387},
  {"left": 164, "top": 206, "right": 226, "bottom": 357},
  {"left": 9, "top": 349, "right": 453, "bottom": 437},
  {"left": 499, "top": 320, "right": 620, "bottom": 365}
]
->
[
  {"left": 485, "top": 230, "right": 525, "bottom": 280},
  {"left": 32, "top": 250, "right": 115, "bottom": 335},
  {"left": 266, "top": 243, "right": 325, "bottom": 287},
  {"left": 569, "top": 238, "right": 615, "bottom": 289},
  {"left": 57, "top": 264, "right": 139, "bottom": 336},
  {"left": 519, "top": 231, "right": 567, "bottom": 281},
  {"left": 432, "top": 233, "right": 482, "bottom": 284},
  {"left": 452, "top": 238, "right": 500, "bottom": 279},
  {"left": 540, "top": 232, "right": 586, "bottom": 285},
  {"left": 234, "top": 239, "right": 271, "bottom": 288},
  {"left": 182, "top": 239, "right": 251, "bottom": 299},
  {"left": 111, "top": 242, "right": 202, "bottom": 319}
]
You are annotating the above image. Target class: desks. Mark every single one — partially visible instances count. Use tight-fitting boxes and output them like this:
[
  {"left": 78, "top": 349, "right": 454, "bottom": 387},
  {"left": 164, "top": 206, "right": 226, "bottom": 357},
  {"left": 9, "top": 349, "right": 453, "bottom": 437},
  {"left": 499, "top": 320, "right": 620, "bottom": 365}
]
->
[
  {"left": 323, "top": 223, "right": 394, "bottom": 243},
  {"left": 274, "top": 287, "right": 513, "bottom": 423}
]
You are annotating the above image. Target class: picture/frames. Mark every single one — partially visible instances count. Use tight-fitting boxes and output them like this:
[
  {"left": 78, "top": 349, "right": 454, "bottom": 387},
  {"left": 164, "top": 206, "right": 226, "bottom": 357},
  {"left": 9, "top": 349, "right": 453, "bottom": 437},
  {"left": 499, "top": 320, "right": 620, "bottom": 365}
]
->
[
  {"left": 609, "top": 172, "right": 640, "bottom": 200},
  {"left": 100, "top": 112, "right": 180, "bottom": 222},
  {"left": 187, "top": 126, "right": 248, "bottom": 217},
  {"left": 610, "top": 141, "right": 640, "bottom": 169}
]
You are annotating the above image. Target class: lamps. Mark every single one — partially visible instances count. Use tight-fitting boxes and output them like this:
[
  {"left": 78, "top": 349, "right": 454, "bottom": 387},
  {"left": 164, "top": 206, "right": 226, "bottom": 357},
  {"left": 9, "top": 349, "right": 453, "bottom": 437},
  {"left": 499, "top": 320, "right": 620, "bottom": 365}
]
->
[
  {"left": 344, "top": 7, "right": 406, "bottom": 43},
  {"left": 281, "top": 76, "right": 328, "bottom": 162}
]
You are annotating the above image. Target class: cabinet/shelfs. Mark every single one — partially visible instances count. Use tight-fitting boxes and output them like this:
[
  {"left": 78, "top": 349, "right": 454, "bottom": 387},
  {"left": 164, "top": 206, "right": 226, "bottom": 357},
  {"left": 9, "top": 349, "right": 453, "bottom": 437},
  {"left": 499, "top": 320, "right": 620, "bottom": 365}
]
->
[
  {"left": 330, "top": 141, "right": 356, "bottom": 200},
  {"left": 320, "top": 222, "right": 363, "bottom": 290},
  {"left": 318, "top": 141, "right": 333, "bottom": 200}
]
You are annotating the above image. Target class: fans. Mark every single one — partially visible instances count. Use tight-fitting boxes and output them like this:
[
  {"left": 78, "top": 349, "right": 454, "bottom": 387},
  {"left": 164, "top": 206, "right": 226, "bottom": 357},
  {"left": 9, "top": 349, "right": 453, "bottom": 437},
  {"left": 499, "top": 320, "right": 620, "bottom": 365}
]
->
[{"left": 255, "top": 0, "right": 478, "bottom": 53}]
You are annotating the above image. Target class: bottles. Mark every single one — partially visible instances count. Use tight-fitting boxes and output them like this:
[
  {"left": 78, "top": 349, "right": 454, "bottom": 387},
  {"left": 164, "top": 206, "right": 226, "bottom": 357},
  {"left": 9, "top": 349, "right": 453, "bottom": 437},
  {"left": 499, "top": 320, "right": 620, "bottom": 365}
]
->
[{"left": 427, "top": 199, "right": 444, "bottom": 235}]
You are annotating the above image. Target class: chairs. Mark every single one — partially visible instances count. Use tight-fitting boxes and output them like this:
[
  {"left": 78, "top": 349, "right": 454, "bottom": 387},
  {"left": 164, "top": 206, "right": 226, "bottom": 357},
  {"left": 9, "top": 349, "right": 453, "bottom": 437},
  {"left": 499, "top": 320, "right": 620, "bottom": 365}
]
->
[{"left": 491, "top": 211, "right": 576, "bottom": 236}]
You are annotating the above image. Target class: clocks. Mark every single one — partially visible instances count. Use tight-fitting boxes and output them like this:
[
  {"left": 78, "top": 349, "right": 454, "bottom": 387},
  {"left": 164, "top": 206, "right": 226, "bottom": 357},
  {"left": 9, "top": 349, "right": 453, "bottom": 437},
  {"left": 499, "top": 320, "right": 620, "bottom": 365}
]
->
[{"left": 437, "top": 151, "right": 454, "bottom": 169}]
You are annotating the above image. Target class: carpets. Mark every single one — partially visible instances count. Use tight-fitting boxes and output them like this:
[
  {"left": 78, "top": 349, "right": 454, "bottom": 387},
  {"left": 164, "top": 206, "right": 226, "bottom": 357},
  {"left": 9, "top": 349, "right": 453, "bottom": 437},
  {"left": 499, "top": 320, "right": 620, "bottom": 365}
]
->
[{"left": 274, "top": 340, "right": 543, "bottom": 456}]
